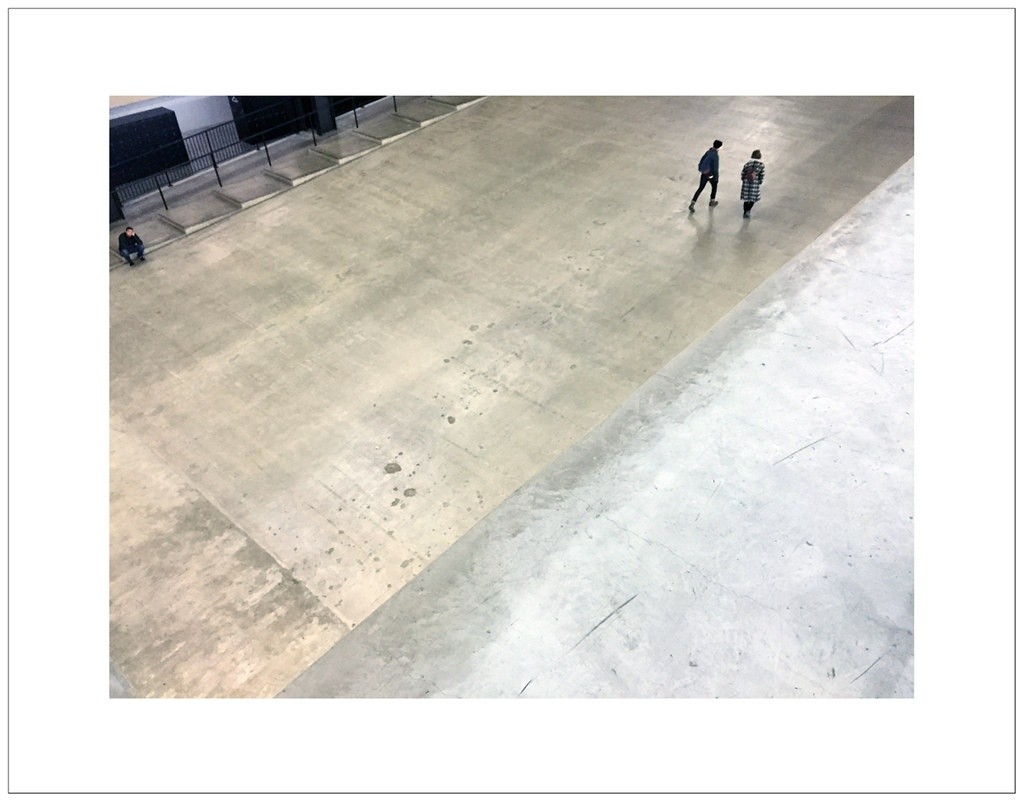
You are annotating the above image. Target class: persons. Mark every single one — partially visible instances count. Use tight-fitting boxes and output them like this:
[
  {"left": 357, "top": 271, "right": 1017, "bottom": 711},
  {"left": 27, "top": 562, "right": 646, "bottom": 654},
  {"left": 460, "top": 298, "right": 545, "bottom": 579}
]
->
[
  {"left": 688, "top": 140, "right": 724, "bottom": 213},
  {"left": 118, "top": 227, "right": 145, "bottom": 266},
  {"left": 740, "top": 149, "right": 766, "bottom": 218}
]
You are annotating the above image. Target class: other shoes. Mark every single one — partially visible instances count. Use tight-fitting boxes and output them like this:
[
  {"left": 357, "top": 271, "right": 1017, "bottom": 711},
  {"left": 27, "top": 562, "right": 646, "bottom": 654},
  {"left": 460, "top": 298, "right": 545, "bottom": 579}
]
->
[
  {"left": 746, "top": 210, "right": 750, "bottom": 217},
  {"left": 743, "top": 215, "right": 749, "bottom": 218},
  {"left": 709, "top": 201, "right": 718, "bottom": 206},
  {"left": 130, "top": 262, "right": 134, "bottom": 266},
  {"left": 689, "top": 202, "right": 695, "bottom": 212},
  {"left": 139, "top": 256, "right": 145, "bottom": 261}
]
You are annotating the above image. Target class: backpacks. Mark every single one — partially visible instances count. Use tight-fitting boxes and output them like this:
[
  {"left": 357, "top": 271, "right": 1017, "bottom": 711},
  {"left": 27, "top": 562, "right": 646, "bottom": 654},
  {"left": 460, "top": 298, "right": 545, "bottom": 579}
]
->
[{"left": 747, "top": 163, "right": 760, "bottom": 183}]
